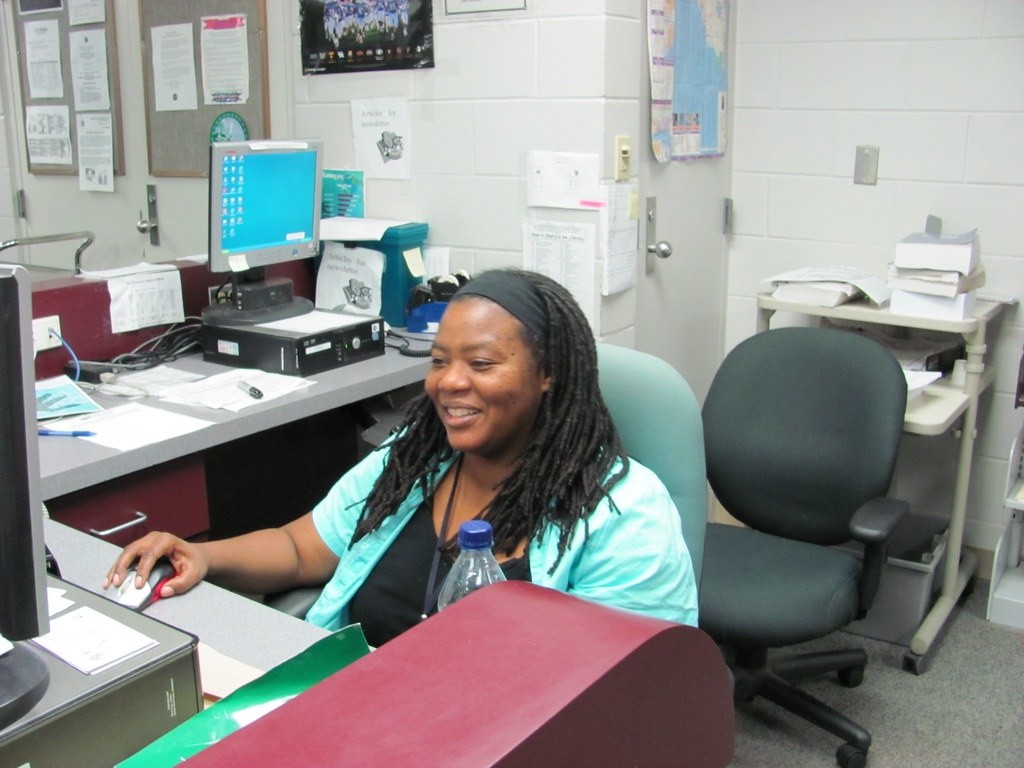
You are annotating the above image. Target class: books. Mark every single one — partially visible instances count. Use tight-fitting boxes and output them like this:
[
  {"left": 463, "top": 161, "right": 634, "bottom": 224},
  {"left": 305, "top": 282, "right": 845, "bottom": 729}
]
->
[{"left": 887, "top": 229, "right": 986, "bottom": 320}]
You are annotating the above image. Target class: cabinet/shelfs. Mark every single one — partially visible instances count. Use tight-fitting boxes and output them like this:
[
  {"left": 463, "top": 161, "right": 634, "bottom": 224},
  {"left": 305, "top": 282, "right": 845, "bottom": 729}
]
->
[
  {"left": 986, "top": 419, "right": 1024, "bottom": 629},
  {"left": 748, "top": 272, "right": 1020, "bottom": 676},
  {"left": 27, "top": 448, "right": 212, "bottom": 589}
]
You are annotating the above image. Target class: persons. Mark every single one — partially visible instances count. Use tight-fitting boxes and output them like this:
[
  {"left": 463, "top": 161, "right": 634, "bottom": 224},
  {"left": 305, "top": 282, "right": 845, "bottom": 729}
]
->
[
  {"left": 323, "top": 0, "right": 409, "bottom": 49},
  {"left": 102, "top": 269, "right": 700, "bottom": 649}
]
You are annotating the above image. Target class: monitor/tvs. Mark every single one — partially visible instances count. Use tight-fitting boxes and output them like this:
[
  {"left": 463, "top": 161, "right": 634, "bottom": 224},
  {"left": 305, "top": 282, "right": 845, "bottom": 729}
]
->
[
  {"left": 201, "top": 138, "right": 323, "bottom": 325},
  {"left": 0, "top": 264, "right": 49, "bottom": 731}
]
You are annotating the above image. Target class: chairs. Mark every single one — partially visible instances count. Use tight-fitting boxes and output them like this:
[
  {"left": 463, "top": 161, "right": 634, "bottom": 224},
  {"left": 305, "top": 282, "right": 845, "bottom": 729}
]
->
[
  {"left": 445, "top": 345, "right": 709, "bottom": 632},
  {"left": 692, "top": 326, "right": 910, "bottom": 768}
]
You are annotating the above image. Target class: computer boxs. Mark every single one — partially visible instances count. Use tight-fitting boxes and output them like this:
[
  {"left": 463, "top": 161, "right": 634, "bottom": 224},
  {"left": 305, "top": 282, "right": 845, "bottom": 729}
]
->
[
  {"left": 0, "top": 572, "right": 205, "bottom": 768},
  {"left": 203, "top": 308, "right": 384, "bottom": 378}
]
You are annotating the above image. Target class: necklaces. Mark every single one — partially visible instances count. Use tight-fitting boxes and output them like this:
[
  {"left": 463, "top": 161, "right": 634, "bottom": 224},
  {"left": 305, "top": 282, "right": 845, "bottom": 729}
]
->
[{"left": 420, "top": 453, "right": 465, "bottom": 623}]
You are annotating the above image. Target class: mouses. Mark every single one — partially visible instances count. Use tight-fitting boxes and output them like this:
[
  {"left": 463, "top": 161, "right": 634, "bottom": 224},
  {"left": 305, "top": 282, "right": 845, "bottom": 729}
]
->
[{"left": 106, "top": 558, "right": 176, "bottom": 612}]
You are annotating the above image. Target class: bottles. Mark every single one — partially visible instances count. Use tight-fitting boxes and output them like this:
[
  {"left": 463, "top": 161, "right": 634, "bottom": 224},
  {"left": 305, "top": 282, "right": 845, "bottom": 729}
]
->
[{"left": 437, "top": 520, "right": 507, "bottom": 614}]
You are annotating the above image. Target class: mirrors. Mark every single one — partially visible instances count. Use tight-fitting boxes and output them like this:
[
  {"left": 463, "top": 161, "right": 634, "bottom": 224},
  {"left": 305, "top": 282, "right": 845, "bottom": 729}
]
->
[{"left": 1, "top": 1, "right": 271, "bottom": 279}]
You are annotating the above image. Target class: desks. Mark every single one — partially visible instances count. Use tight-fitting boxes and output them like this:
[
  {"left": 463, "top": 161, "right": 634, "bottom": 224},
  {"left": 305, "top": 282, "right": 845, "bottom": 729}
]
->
[
  {"left": 22, "top": 502, "right": 384, "bottom": 768},
  {"left": 37, "top": 299, "right": 528, "bottom": 507}
]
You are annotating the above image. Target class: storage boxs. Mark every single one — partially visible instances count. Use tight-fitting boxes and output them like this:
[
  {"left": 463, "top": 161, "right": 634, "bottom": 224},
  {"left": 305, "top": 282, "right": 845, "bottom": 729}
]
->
[{"left": 837, "top": 510, "right": 956, "bottom": 650}]
